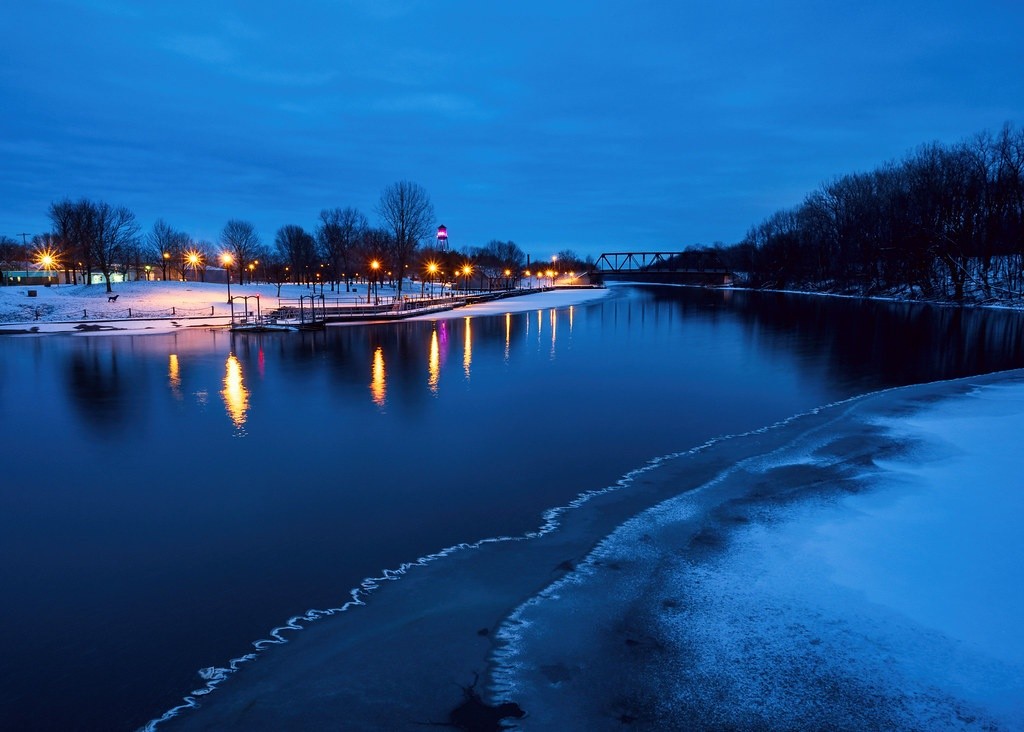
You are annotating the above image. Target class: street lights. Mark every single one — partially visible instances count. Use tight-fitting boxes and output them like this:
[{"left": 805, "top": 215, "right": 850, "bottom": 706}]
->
[{"left": 37, "top": 256, "right": 555, "bottom": 305}]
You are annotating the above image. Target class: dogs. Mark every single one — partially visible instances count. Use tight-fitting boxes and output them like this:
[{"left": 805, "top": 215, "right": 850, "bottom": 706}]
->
[{"left": 107, "top": 295, "right": 119, "bottom": 302}]
